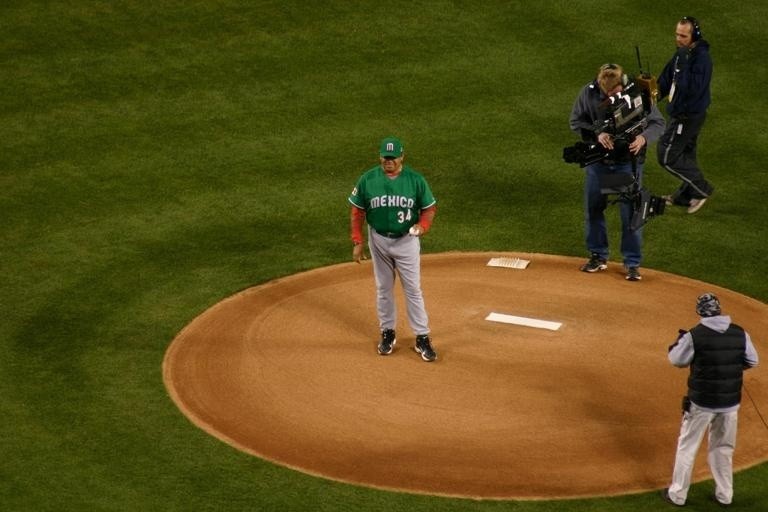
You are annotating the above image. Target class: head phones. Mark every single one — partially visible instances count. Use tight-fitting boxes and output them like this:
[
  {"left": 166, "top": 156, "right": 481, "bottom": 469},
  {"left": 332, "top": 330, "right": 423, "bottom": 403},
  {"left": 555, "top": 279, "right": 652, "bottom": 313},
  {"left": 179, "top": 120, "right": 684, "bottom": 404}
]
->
[{"left": 682, "top": 16, "right": 701, "bottom": 42}]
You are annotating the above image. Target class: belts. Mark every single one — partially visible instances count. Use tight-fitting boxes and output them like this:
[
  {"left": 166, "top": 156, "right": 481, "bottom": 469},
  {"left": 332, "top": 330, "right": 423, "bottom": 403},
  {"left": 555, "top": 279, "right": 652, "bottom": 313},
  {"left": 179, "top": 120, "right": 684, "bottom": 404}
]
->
[{"left": 376, "top": 230, "right": 409, "bottom": 238}]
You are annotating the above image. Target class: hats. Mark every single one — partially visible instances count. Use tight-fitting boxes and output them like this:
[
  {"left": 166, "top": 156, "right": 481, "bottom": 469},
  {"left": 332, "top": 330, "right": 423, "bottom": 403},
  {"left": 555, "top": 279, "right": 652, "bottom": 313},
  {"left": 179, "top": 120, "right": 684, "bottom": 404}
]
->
[
  {"left": 696, "top": 293, "right": 721, "bottom": 316},
  {"left": 379, "top": 138, "right": 403, "bottom": 158}
]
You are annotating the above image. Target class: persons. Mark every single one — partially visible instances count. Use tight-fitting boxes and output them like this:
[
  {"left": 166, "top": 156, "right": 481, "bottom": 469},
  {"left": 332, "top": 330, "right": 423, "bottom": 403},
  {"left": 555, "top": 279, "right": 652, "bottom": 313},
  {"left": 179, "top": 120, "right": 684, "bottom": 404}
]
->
[
  {"left": 655, "top": 16, "right": 714, "bottom": 214},
  {"left": 569, "top": 64, "right": 666, "bottom": 281},
  {"left": 348, "top": 137, "right": 439, "bottom": 362},
  {"left": 662, "top": 292, "right": 759, "bottom": 507}
]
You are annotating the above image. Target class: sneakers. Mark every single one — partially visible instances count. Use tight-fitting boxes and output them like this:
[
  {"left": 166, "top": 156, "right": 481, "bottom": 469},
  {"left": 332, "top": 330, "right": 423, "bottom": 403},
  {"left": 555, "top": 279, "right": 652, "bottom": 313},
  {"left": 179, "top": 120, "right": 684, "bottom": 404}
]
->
[
  {"left": 581, "top": 257, "right": 608, "bottom": 272},
  {"left": 687, "top": 199, "right": 706, "bottom": 214},
  {"left": 415, "top": 335, "right": 437, "bottom": 361},
  {"left": 661, "top": 195, "right": 672, "bottom": 205},
  {"left": 625, "top": 267, "right": 641, "bottom": 280},
  {"left": 378, "top": 329, "right": 396, "bottom": 354}
]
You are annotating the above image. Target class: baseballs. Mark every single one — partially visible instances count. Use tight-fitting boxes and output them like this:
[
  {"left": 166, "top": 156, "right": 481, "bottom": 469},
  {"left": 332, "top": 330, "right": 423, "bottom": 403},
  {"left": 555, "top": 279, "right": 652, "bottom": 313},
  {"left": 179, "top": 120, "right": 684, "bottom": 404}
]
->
[{"left": 409, "top": 227, "right": 419, "bottom": 236}]
